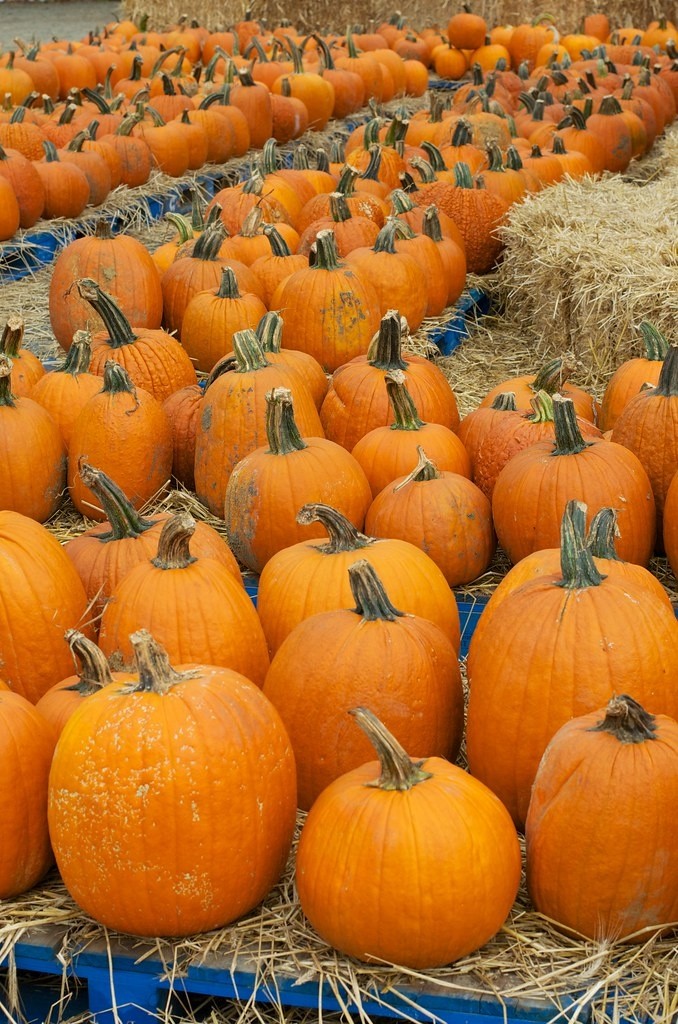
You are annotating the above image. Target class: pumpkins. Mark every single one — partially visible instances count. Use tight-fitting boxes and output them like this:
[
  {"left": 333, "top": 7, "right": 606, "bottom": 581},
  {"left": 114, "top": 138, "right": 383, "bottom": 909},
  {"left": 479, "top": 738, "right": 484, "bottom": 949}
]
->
[{"left": 0, "top": 4, "right": 678, "bottom": 966}]
